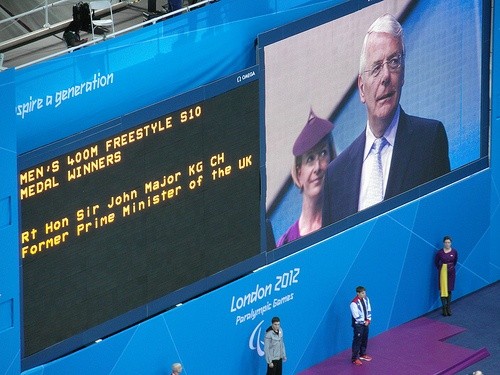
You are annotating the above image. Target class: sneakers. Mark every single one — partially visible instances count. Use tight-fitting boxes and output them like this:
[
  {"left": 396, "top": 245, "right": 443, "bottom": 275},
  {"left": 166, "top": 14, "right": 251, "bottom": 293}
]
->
[{"left": 353, "top": 354, "right": 371, "bottom": 365}]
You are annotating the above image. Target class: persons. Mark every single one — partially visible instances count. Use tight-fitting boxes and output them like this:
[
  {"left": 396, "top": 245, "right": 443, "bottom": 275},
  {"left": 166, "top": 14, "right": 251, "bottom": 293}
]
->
[
  {"left": 265, "top": 317, "right": 287, "bottom": 375},
  {"left": 171, "top": 363, "right": 183, "bottom": 375},
  {"left": 349, "top": 286, "right": 372, "bottom": 366},
  {"left": 275, "top": 13, "right": 449, "bottom": 248},
  {"left": 435, "top": 236, "right": 459, "bottom": 317}
]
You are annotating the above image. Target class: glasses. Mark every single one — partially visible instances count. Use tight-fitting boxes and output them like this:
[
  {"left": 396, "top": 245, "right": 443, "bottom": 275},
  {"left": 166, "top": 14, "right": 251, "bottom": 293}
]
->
[{"left": 362, "top": 56, "right": 404, "bottom": 77}]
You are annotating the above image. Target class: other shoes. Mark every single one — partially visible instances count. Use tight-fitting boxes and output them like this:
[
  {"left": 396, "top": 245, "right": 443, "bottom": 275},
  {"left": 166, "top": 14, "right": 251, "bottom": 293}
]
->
[{"left": 442, "top": 309, "right": 452, "bottom": 317}]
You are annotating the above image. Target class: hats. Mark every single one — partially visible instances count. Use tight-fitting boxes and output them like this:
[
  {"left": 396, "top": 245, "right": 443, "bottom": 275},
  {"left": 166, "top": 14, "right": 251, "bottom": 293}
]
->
[{"left": 293, "top": 116, "right": 334, "bottom": 156}]
